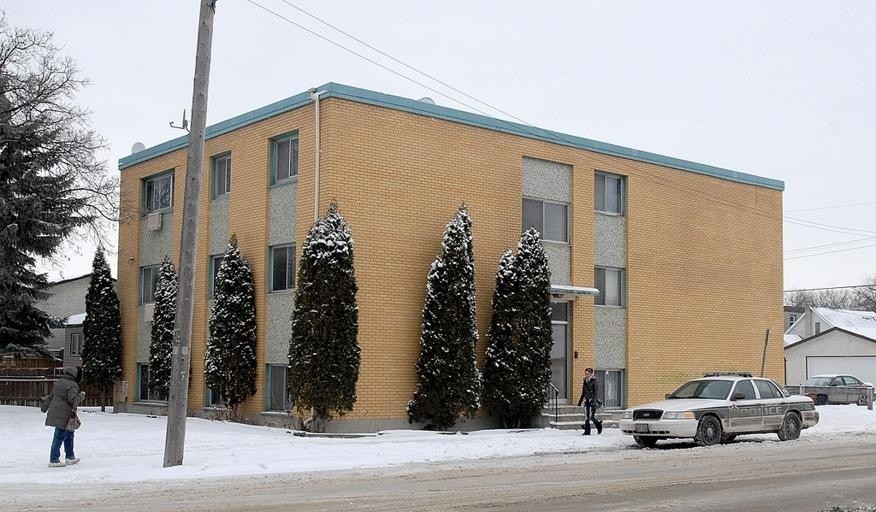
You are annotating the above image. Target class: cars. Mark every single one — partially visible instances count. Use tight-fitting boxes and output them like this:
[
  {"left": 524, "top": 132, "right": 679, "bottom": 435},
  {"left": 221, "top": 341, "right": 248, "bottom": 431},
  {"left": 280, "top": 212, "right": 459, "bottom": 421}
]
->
[
  {"left": 617, "top": 369, "right": 822, "bottom": 450},
  {"left": 796, "top": 371, "right": 876, "bottom": 406}
]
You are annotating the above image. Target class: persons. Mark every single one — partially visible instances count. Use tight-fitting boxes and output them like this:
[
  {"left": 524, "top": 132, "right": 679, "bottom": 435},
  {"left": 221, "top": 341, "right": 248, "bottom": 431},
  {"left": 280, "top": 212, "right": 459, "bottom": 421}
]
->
[
  {"left": 45, "top": 366, "right": 86, "bottom": 467},
  {"left": 578, "top": 368, "right": 603, "bottom": 435}
]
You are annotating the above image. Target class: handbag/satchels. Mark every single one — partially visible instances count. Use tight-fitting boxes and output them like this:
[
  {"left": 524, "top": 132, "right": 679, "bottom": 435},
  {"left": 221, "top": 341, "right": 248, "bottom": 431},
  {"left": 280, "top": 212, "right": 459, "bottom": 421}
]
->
[
  {"left": 589, "top": 398, "right": 602, "bottom": 410},
  {"left": 65, "top": 396, "right": 81, "bottom": 433}
]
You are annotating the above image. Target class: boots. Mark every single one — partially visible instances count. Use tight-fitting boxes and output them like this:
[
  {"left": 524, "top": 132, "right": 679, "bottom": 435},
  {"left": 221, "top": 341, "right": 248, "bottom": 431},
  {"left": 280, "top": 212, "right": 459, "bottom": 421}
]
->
[
  {"left": 581, "top": 424, "right": 590, "bottom": 435},
  {"left": 594, "top": 420, "right": 602, "bottom": 434}
]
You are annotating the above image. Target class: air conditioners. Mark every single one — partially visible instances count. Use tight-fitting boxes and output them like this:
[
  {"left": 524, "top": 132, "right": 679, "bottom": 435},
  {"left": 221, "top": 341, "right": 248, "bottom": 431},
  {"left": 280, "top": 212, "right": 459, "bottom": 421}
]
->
[
  {"left": 144, "top": 304, "right": 155, "bottom": 322},
  {"left": 147, "top": 212, "right": 162, "bottom": 231}
]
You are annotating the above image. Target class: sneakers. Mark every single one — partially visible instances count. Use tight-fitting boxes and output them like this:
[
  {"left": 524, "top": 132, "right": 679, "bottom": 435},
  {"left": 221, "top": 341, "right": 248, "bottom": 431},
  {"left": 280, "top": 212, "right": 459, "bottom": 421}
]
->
[
  {"left": 49, "top": 462, "right": 66, "bottom": 467},
  {"left": 66, "top": 457, "right": 80, "bottom": 465}
]
